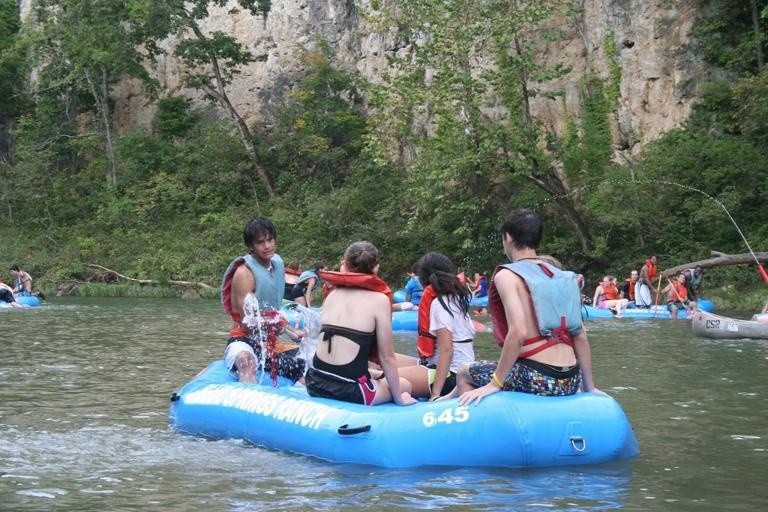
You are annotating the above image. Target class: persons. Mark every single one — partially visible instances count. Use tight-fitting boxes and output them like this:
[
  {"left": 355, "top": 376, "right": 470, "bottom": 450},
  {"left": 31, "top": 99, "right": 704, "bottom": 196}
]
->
[
  {"left": 456, "top": 266, "right": 489, "bottom": 298},
  {"left": 0, "top": 280, "right": 19, "bottom": 308},
  {"left": 10, "top": 265, "right": 45, "bottom": 301},
  {"left": 391, "top": 262, "right": 423, "bottom": 311},
  {"left": 455, "top": 211, "right": 595, "bottom": 407},
  {"left": 282, "top": 261, "right": 302, "bottom": 300},
  {"left": 577, "top": 255, "right": 703, "bottom": 318},
  {"left": 752, "top": 303, "right": 768, "bottom": 321},
  {"left": 221, "top": 216, "right": 304, "bottom": 383},
  {"left": 395, "top": 253, "right": 476, "bottom": 403},
  {"left": 304, "top": 240, "right": 418, "bottom": 407},
  {"left": 292, "top": 262, "right": 327, "bottom": 307}
]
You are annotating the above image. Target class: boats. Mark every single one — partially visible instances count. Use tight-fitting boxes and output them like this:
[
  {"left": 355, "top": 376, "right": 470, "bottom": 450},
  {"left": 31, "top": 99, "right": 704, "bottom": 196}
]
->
[
  {"left": 692, "top": 309, "right": 768, "bottom": 338},
  {"left": 393, "top": 290, "right": 491, "bottom": 307},
  {"left": 282, "top": 304, "right": 423, "bottom": 331},
  {"left": 583, "top": 299, "right": 712, "bottom": 321},
  {"left": 3, "top": 294, "right": 37, "bottom": 307},
  {"left": 168, "top": 355, "right": 641, "bottom": 470}
]
